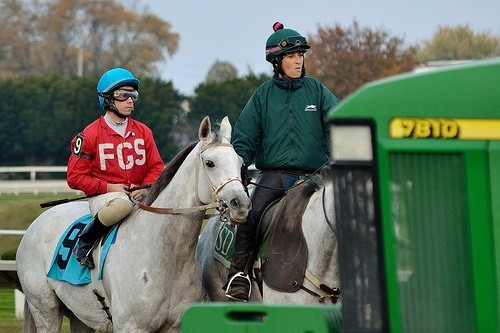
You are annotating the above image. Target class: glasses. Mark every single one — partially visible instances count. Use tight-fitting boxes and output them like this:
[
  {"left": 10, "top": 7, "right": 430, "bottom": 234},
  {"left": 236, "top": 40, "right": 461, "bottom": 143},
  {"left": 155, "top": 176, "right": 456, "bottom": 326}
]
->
[{"left": 97, "top": 88, "right": 139, "bottom": 103}]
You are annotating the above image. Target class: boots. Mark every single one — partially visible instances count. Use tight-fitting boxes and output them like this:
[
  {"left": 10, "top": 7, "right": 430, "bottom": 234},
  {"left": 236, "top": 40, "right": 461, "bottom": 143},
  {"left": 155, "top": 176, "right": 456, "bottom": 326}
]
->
[
  {"left": 74, "top": 212, "right": 111, "bottom": 269},
  {"left": 225, "top": 231, "right": 261, "bottom": 299}
]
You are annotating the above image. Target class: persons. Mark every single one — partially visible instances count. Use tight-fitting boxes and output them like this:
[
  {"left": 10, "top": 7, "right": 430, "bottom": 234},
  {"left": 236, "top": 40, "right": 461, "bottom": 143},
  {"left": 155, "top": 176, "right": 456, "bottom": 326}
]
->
[
  {"left": 67, "top": 69, "right": 165, "bottom": 269},
  {"left": 221, "top": 22, "right": 337, "bottom": 300}
]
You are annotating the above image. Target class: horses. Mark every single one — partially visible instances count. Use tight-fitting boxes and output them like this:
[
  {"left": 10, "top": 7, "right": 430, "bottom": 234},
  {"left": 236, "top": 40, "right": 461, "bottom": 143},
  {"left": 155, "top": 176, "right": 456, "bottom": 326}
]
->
[
  {"left": 197, "top": 166, "right": 341, "bottom": 304},
  {"left": 15, "top": 116, "right": 252, "bottom": 333}
]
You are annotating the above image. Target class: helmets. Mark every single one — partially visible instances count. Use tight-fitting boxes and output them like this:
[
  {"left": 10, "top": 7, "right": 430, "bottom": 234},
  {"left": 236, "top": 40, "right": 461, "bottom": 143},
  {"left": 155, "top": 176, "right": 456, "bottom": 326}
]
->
[
  {"left": 265, "top": 21, "right": 310, "bottom": 61},
  {"left": 96, "top": 67, "right": 140, "bottom": 108}
]
